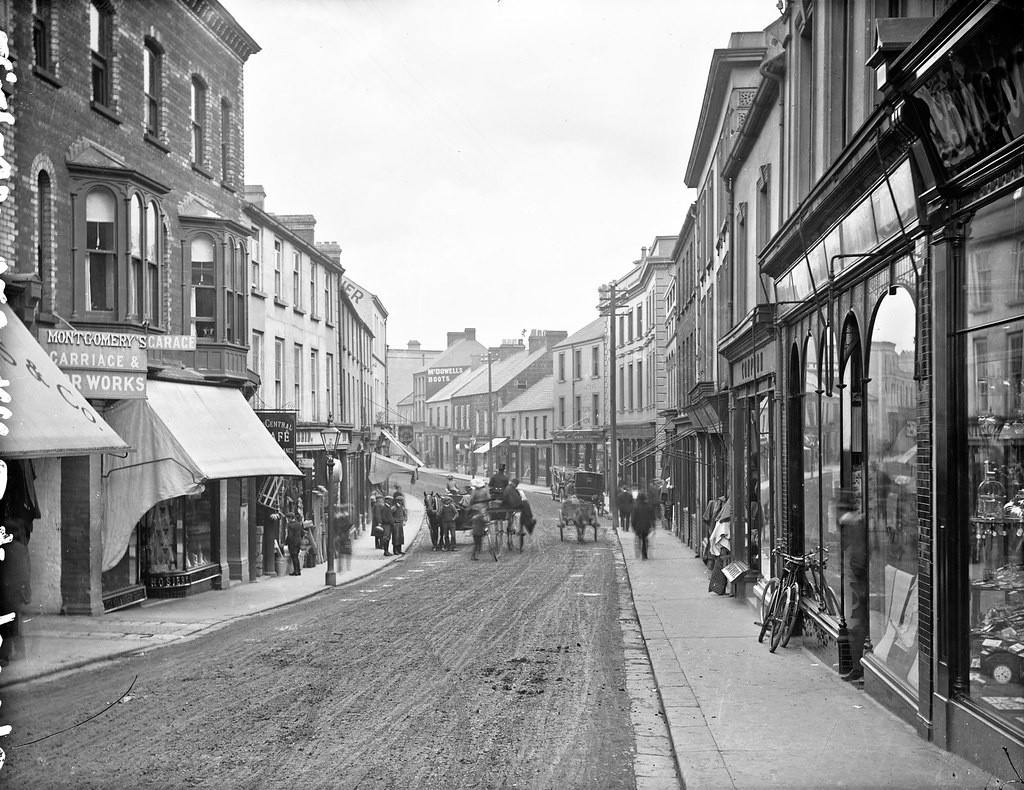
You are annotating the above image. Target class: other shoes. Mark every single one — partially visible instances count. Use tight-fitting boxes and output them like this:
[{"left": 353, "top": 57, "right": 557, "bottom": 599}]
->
[
  {"left": 289, "top": 572, "right": 298, "bottom": 575},
  {"left": 298, "top": 571, "right": 301, "bottom": 575}
]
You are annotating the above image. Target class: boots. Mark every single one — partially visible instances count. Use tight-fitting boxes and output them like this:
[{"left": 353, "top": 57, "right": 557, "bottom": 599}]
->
[
  {"left": 375, "top": 540, "right": 383, "bottom": 549},
  {"left": 383, "top": 541, "right": 392, "bottom": 556},
  {"left": 380, "top": 539, "right": 384, "bottom": 547},
  {"left": 393, "top": 545, "right": 399, "bottom": 555},
  {"left": 398, "top": 544, "right": 405, "bottom": 554}
]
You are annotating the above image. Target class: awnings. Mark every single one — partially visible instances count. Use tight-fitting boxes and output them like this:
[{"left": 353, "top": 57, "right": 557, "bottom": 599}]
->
[
  {"left": 368, "top": 451, "right": 420, "bottom": 484},
  {"left": 0, "top": 283, "right": 138, "bottom": 458},
  {"left": 380, "top": 427, "right": 425, "bottom": 467},
  {"left": 471, "top": 437, "right": 509, "bottom": 454},
  {"left": 98, "top": 377, "right": 307, "bottom": 572}
]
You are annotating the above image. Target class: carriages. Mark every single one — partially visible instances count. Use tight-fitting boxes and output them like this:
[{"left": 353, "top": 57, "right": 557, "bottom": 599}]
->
[
  {"left": 558, "top": 497, "right": 600, "bottom": 542},
  {"left": 423, "top": 486, "right": 528, "bottom": 552}
]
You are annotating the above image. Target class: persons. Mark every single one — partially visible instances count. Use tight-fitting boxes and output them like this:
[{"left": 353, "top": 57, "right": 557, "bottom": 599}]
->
[
  {"left": 280, "top": 511, "right": 305, "bottom": 576},
  {"left": 330, "top": 480, "right": 408, "bottom": 571},
  {"left": 0, "top": 512, "right": 40, "bottom": 668},
  {"left": 430, "top": 462, "right": 536, "bottom": 561},
  {"left": 561, "top": 465, "right": 661, "bottom": 561}
]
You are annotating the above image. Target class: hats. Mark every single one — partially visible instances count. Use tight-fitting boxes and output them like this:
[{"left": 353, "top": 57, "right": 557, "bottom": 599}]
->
[
  {"left": 471, "top": 478, "right": 485, "bottom": 488},
  {"left": 499, "top": 463, "right": 506, "bottom": 471},
  {"left": 384, "top": 495, "right": 394, "bottom": 499},
  {"left": 442, "top": 497, "right": 451, "bottom": 502},
  {"left": 375, "top": 495, "right": 383, "bottom": 498},
  {"left": 396, "top": 496, "right": 404, "bottom": 499},
  {"left": 286, "top": 512, "right": 295, "bottom": 517},
  {"left": 510, "top": 479, "right": 520, "bottom": 484}
]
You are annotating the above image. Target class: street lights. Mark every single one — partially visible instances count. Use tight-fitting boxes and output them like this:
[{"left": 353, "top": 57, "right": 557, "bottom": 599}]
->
[
  {"left": 320, "top": 409, "right": 341, "bottom": 585},
  {"left": 470, "top": 434, "right": 476, "bottom": 479},
  {"left": 596, "top": 280, "right": 631, "bottom": 527},
  {"left": 481, "top": 352, "right": 498, "bottom": 478}
]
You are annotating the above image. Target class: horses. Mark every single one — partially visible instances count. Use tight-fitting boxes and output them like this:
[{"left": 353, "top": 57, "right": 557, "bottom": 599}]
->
[
  {"left": 573, "top": 500, "right": 590, "bottom": 544},
  {"left": 423, "top": 490, "right": 445, "bottom": 551}
]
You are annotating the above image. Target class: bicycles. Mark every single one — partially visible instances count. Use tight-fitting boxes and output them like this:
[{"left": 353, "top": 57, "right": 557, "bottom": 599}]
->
[
  {"left": 479, "top": 505, "right": 499, "bottom": 562},
  {"left": 754, "top": 546, "right": 829, "bottom": 652}
]
